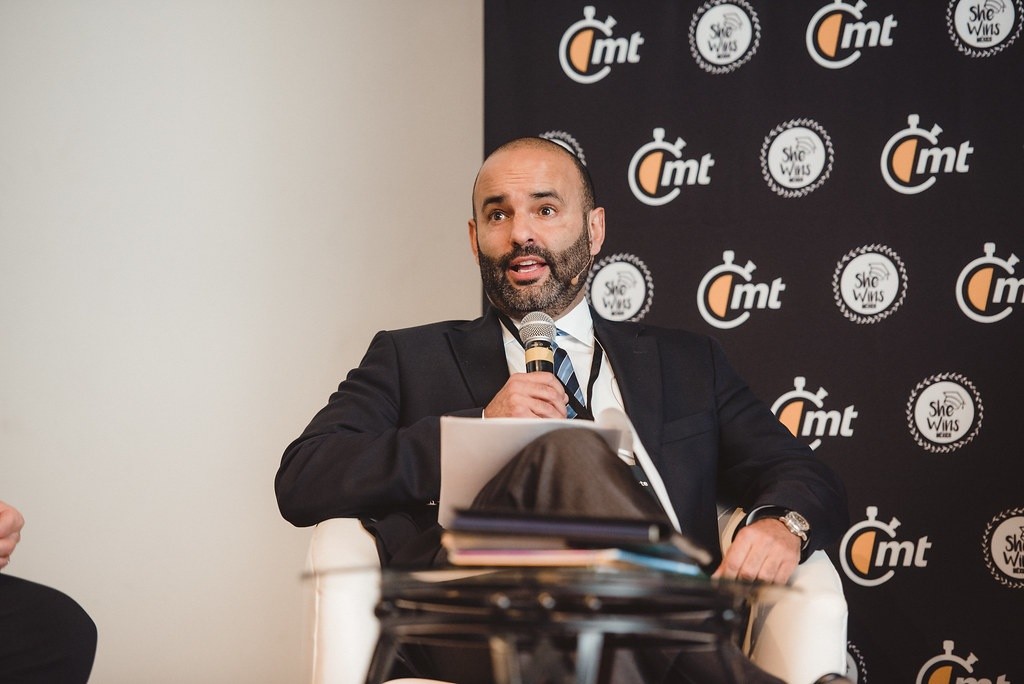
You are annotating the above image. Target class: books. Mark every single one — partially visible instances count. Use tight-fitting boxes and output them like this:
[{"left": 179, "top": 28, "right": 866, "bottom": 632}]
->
[{"left": 388, "top": 507, "right": 736, "bottom": 649}]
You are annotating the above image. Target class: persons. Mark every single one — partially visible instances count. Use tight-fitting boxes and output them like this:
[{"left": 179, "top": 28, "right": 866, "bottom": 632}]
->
[
  {"left": 0, "top": 497, "right": 100, "bottom": 684},
  {"left": 272, "top": 135, "right": 853, "bottom": 684}
]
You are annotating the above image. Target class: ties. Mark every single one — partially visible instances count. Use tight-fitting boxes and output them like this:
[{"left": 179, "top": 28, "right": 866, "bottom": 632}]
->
[{"left": 553, "top": 328, "right": 587, "bottom": 420}]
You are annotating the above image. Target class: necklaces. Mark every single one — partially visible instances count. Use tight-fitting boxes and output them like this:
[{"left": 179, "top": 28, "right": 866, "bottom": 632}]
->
[{"left": 494, "top": 309, "right": 602, "bottom": 422}]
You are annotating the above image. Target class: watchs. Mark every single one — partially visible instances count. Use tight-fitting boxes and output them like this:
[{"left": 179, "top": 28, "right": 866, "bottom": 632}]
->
[{"left": 753, "top": 508, "right": 813, "bottom": 553}]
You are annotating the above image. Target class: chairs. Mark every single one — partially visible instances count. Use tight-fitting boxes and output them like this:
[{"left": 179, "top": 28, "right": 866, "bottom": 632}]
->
[{"left": 302, "top": 515, "right": 850, "bottom": 684}]
